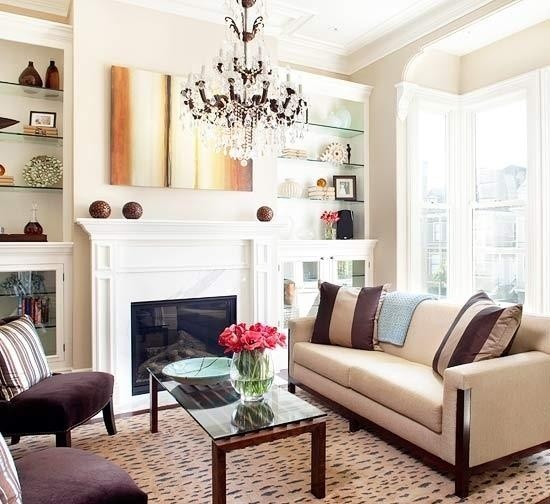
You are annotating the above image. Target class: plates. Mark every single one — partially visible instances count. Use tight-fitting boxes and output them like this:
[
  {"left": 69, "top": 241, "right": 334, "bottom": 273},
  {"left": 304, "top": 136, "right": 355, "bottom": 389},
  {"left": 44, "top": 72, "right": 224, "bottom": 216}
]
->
[{"left": 162, "top": 355, "right": 235, "bottom": 385}]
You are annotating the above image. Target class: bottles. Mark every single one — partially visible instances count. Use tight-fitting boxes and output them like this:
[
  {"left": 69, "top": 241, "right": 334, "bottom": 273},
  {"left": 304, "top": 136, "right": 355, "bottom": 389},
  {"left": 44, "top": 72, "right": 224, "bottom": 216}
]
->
[
  {"left": 45, "top": 60, "right": 60, "bottom": 90},
  {"left": 18, "top": 61, "right": 43, "bottom": 88},
  {"left": 24, "top": 200, "right": 43, "bottom": 237}
]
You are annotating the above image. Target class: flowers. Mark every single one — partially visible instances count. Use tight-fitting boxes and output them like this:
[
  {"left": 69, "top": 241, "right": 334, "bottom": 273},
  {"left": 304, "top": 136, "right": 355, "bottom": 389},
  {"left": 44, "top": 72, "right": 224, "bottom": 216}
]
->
[
  {"left": 218, "top": 319, "right": 287, "bottom": 353},
  {"left": 320, "top": 211, "right": 339, "bottom": 226}
]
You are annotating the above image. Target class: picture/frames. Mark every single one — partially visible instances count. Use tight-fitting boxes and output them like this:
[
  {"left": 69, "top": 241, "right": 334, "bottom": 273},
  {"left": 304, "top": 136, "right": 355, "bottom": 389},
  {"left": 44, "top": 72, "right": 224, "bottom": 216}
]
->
[
  {"left": 29, "top": 110, "right": 56, "bottom": 128},
  {"left": 333, "top": 174, "right": 357, "bottom": 200}
]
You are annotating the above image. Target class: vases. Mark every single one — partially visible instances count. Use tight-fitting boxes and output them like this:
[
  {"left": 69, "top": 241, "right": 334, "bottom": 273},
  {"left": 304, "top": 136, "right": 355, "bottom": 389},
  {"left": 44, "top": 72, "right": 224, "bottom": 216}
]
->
[
  {"left": 323, "top": 226, "right": 337, "bottom": 240},
  {"left": 229, "top": 350, "right": 275, "bottom": 430}
]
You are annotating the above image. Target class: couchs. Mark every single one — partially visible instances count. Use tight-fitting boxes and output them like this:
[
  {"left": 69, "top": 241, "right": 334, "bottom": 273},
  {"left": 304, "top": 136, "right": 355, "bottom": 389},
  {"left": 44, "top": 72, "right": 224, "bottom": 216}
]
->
[
  {"left": 0, "top": 433, "right": 148, "bottom": 504},
  {"left": 1, "top": 315, "right": 117, "bottom": 447},
  {"left": 286, "top": 292, "right": 550, "bottom": 498}
]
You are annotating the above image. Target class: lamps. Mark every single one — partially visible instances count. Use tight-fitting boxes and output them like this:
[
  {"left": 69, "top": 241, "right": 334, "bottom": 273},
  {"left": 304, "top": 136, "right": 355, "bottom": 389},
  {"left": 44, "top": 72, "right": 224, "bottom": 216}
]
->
[{"left": 178, "top": 0, "right": 309, "bottom": 169}]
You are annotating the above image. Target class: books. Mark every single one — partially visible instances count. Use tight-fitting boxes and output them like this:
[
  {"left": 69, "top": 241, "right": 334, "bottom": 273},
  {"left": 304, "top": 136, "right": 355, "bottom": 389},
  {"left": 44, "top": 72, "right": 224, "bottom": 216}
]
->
[{"left": 18, "top": 295, "right": 50, "bottom": 325}]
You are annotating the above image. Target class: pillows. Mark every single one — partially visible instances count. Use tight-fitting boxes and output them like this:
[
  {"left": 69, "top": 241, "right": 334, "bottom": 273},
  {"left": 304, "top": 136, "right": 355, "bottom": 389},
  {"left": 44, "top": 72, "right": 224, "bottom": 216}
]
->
[
  {"left": 310, "top": 282, "right": 390, "bottom": 352},
  {"left": 432, "top": 290, "right": 523, "bottom": 375}
]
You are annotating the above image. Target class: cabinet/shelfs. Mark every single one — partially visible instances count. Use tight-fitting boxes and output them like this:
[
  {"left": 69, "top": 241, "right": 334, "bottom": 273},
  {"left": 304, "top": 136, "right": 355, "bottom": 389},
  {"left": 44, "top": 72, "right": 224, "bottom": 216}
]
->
[
  {"left": 1, "top": 244, "right": 73, "bottom": 372},
  {"left": 262, "top": 90, "right": 372, "bottom": 239},
  {"left": 0, "top": 12, "right": 74, "bottom": 242},
  {"left": 267, "top": 241, "right": 377, "bottom": 370}
]
